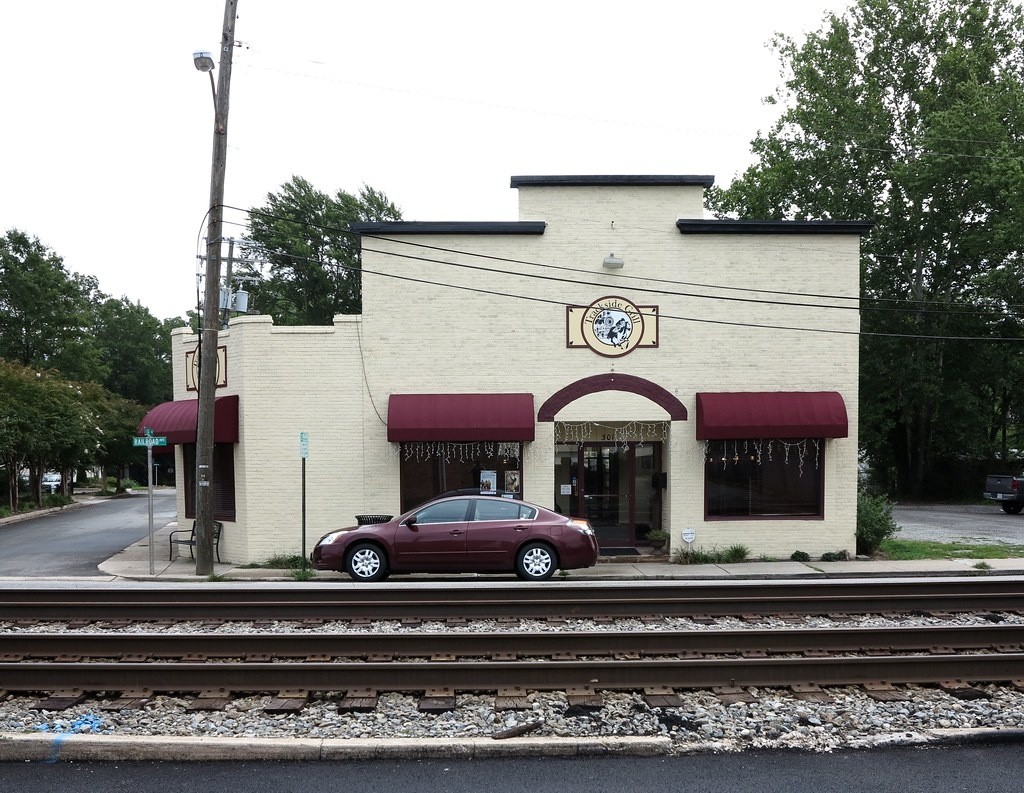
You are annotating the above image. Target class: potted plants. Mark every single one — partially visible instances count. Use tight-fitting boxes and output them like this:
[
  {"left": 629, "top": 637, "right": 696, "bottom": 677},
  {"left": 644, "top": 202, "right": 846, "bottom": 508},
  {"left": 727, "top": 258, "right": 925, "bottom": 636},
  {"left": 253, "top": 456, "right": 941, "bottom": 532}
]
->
[{"left": 644, "top": 530, "right": 670, "bottom": 556}]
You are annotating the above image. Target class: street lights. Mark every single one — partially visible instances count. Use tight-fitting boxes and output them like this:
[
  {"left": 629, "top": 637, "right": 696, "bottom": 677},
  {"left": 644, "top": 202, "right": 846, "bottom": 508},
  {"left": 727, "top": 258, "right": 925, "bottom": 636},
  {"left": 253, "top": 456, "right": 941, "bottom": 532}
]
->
[{"left": 192, "top": 51, "right": 233, "bottom": 576}]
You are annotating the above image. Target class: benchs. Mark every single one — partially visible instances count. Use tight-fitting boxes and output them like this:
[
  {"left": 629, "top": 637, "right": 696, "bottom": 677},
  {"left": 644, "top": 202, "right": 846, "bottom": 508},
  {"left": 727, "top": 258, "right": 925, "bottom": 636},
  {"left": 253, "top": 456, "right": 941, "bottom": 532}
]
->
[{"left": 169, "top": 520, "right": 222, "bottom": 564}]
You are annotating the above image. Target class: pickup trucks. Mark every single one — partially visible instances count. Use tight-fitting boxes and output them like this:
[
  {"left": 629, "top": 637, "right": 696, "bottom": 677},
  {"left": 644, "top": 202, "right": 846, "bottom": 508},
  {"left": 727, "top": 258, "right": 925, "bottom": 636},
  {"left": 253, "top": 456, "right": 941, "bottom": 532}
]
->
[{"left": 982, "top": 472, "right": 1024, "bottom": 514}]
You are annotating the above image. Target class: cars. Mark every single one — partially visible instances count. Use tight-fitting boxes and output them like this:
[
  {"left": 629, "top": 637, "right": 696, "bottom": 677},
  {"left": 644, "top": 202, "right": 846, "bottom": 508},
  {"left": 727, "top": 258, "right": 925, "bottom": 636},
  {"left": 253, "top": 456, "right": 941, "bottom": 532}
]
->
[
  {"left": 310, "top": 495, "right": 599, "bottom": 581},
  {"left": 41, "top": 472, "right": 62, "bottom": 493},
  {"left": 19, "top": 468, "right": 30, "bottom": 486}
]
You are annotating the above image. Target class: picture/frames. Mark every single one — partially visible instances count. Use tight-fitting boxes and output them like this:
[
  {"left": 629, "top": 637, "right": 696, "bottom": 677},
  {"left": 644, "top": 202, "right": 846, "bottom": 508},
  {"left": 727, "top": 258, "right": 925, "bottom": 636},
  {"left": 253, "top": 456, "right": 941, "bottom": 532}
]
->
[{"left": 641, "top": 457, "right": 651, "bottom": 469}]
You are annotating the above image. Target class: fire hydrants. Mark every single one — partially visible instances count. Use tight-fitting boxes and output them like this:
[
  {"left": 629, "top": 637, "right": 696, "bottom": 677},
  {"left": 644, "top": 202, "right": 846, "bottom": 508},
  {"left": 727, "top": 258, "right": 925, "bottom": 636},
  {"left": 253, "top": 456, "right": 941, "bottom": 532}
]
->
[{"left": 51, "top": 483, "right": 56, "bottom": 495}]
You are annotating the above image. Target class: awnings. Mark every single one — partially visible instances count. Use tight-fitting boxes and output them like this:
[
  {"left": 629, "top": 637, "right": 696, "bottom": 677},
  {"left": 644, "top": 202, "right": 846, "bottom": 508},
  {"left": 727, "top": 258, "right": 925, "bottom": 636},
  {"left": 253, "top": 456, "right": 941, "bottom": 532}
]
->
[
  {"left": 695, "top": 392, "right": 848, "bottom": 442},
  {"left": 136, "top": 394, "right": 239, "bottom": 444},
  {"left": 386, "top": 393, "right": 537, "bottom": 446}
]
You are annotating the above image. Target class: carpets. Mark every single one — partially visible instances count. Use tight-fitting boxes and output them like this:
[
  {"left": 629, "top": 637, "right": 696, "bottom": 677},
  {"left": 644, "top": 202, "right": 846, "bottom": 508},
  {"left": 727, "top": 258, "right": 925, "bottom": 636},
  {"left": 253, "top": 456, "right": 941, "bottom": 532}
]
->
[{"left": 599, "top": 547, "right": 641, "bottom": 556}]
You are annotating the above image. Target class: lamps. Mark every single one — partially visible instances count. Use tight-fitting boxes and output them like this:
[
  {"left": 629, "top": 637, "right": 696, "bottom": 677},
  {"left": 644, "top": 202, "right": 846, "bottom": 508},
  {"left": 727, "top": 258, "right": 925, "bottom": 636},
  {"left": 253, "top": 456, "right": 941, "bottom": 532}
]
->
[{"left": 603, "top": 252, "right": 625, "bottom": 269}]
